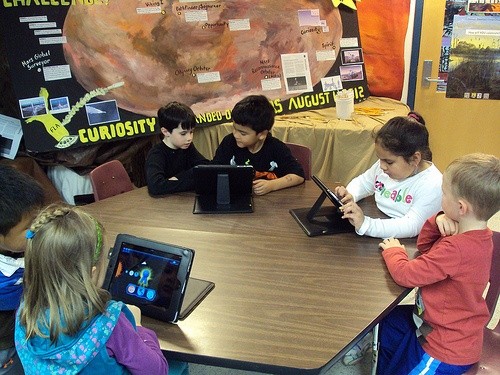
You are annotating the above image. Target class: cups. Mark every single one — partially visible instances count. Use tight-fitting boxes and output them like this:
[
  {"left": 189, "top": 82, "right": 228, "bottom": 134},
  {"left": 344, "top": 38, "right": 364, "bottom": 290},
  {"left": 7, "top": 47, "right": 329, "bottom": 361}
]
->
[{"left": 335, "top": 91, "right": 354, "bottom": 119}]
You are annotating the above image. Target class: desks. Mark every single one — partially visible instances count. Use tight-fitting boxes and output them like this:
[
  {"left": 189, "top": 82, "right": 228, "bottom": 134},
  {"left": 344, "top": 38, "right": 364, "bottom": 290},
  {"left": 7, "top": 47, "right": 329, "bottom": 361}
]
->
[
  {"left": 192, "top": 96, "right": 411, "bottom": 184},
  {"left": 76, "top": 177, "right": 422, "bottom": 375}
]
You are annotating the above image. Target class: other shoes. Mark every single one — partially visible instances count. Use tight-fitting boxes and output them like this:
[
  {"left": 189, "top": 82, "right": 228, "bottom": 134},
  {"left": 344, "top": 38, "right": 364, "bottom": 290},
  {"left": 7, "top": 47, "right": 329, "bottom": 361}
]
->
[{"left": 343, "top": 331, "right": 374, "bottom": 365}]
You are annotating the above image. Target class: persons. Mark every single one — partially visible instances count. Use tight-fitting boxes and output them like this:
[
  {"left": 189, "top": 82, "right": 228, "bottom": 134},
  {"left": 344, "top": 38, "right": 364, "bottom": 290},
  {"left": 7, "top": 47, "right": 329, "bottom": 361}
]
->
[
  {"left": 212, "top": 95, "right": 306, "bottom": 196},
  {"left": 15, "top": 202, "right": 191, "bottom": 375},
  {"left": 144, "top": 101, "right": 212, "bottom": 195},
  {"left": 334, "top": 110, "right": 443, "bottom": 239},
  {"left": 0, "top": 166, "right": 48, "bottom": 375},
  {"left": 376, "top": 153, "right": 500, "bottom": 375}
]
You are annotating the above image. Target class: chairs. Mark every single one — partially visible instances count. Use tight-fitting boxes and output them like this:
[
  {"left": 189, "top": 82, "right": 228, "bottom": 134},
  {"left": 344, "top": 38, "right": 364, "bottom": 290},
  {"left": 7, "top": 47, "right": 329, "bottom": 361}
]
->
[
  {"left": 461, "top": 230, "right": 500, "bottom": 375},
  {"left": 284, "top": 140, "right": 312, "bottom": 181},
  {"left": 89, "top": 160, "right": 134, "bottom": 203}
]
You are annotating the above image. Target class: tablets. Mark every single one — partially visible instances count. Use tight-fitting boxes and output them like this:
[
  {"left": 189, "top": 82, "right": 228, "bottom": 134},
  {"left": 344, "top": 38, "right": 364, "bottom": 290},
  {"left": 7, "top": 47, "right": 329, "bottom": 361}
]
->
[
  {"left": 193, "top": 165, "right": 254, "bottom": 201},
  {"left": 313, "top": 175, "right": 344, "bottom": 206},
  {"left": 102, "top": 234, "right": 194, "bottom": 323}
]
